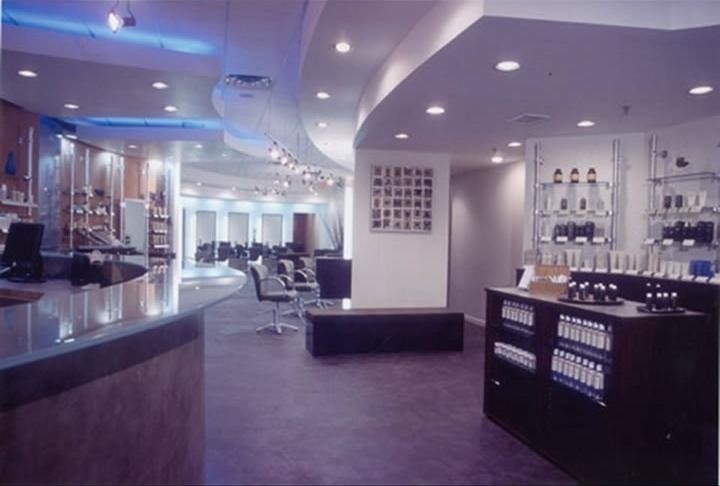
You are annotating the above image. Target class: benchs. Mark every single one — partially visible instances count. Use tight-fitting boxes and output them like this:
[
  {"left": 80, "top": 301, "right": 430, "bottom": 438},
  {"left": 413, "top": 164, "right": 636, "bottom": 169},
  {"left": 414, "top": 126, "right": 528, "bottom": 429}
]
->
[{"left": 300, "top": 306, "right": 465, "bottom": 359}]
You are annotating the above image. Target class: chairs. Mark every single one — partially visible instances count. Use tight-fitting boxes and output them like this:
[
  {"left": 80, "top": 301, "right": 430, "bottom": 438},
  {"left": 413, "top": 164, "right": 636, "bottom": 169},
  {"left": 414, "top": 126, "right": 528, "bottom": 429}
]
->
[{"left": 193, "top": 238, "right": 353, "bottom": 338}]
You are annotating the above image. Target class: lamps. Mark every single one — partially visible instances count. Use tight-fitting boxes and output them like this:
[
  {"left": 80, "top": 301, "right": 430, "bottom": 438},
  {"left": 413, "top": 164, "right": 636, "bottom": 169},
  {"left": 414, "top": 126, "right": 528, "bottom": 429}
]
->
[
  {"left": 105, "top": 0, "right": 138, "bottom": 35},
  {"left": 689, "top": 84, "right": 711, "bottom": 100}
]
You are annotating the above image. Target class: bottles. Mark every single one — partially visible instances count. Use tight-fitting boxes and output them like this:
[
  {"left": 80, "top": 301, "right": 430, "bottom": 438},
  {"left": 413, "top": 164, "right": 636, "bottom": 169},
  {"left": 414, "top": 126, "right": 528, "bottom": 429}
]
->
[
  {"left": 0, "top": 182, "right": 26, "bottom": 203},
  {"left": 565, "top": 281, "right": 619, "bottom": 303},
  {"left": 493, "top": 341, "right": 537, "bottom": 375},
  {"left": 556, "top": 314, "right": 613, "bottom": 362},
  {"left": 569, "top": 168, "right": 581, "bottom": 183},
  {"left": 150, "top": 205, "right": 167, "bottom": 247},
  {"left": 552, "top": 348, "right": 606, "bottom": 398},
  {"left": 585, "top": 168, "right": 598, "bottom": 183},
  {"left": 643, "top": 282, "right": 680, "bottom": 311},
  {"left": 499, "top": 299, "right": 537, "bottom": 337},
  {"left": 552, "top": 168, "right": 563, "bottom": 183},
  {"left": 530, "top": 172, "right": 720, "bottom": 287}
]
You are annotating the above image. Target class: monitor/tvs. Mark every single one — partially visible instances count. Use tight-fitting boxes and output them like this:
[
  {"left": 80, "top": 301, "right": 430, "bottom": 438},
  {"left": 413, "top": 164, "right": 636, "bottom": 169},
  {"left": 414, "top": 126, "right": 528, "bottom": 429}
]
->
[{"left": 3, "top": 222, "right": 44, "bottom": 284}]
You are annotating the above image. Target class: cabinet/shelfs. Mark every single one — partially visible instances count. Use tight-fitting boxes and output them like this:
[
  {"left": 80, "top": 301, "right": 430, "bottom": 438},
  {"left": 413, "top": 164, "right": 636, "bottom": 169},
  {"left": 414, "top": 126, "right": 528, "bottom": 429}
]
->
[{"left": 0, "top": 125, "right": 178, "bottom": 268}]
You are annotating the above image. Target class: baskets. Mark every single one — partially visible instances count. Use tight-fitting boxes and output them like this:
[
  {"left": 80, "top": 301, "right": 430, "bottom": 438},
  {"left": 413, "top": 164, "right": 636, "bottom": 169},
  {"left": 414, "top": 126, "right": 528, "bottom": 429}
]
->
[{"left": 527, "top": 262, "right": 573, "bottom": 297}]
